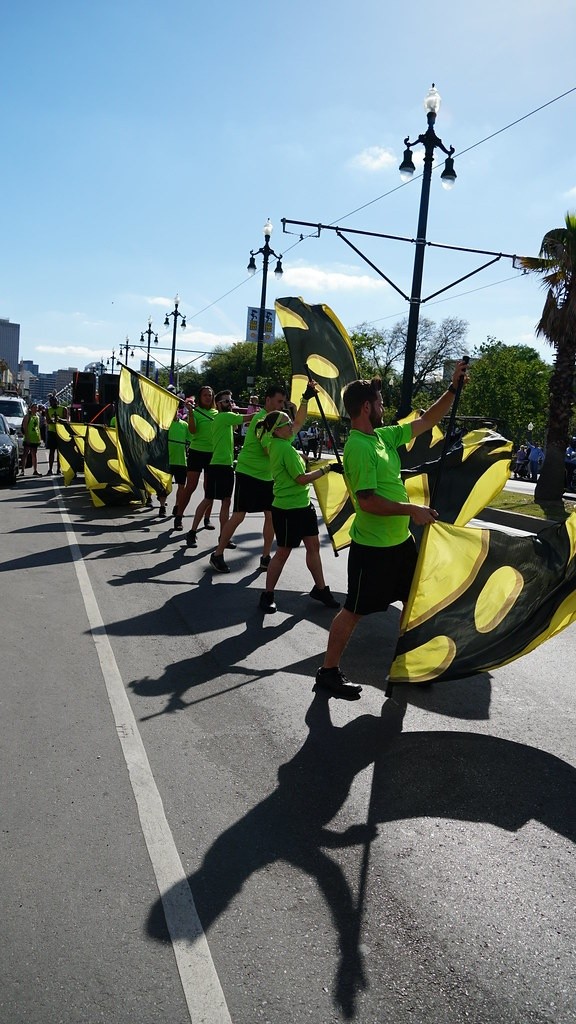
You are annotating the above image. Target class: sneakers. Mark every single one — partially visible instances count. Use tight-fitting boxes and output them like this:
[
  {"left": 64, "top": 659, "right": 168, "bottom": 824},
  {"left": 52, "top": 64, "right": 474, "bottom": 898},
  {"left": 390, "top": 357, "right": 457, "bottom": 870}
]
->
[
  {"left": 210, "top": 552, "right": 229, "bottom": 573},
  {"left": 316, "top": 667, "right": 362, "bottom": 695},
  {"left": 259, "top": 591, "right": 277, "bottom": 613},
  {"left": 309, "top": 584, "right": 341, "bottom": 609},
  {"left": 259, "top": 554, "right": 271, "bottom": 569}
]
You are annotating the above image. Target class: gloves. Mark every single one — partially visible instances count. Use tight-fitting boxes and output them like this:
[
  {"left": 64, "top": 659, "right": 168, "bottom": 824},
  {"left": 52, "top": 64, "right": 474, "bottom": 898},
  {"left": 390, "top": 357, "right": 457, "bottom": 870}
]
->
[{"left": 328, "top": 463, "right": 344, "bottom": 476}]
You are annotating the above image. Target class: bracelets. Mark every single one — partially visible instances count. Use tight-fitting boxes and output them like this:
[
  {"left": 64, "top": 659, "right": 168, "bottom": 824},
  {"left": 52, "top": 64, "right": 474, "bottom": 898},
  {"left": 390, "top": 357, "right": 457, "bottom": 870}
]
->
[{"left": 446, "top": 382, "right": 456, "bottom": 394}]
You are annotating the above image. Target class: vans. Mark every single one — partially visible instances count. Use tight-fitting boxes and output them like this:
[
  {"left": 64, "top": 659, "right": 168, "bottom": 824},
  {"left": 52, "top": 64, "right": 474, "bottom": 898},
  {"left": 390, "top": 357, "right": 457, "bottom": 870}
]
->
[{"left": 241, "top": 421, "right": 253, "bottom": 437}]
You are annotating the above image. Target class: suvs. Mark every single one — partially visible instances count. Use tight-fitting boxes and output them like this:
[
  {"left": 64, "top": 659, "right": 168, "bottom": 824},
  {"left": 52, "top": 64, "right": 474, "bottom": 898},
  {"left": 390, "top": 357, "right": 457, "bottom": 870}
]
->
[{"left": 1, "top": 395, "right": 33, "bottom": 467}]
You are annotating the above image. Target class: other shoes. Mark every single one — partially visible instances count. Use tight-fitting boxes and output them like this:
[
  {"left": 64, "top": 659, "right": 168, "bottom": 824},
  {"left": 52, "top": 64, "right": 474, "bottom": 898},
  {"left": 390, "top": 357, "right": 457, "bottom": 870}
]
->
[
  {"left": 172, "top": 506, "right": 185, "bottom": 517},
  {"left": 18, "top": 472, "right": 24, "bottom": 476},
  {"left": 184, "top": 529, "right": 197, "bottom": 548},
  {"left": 33, "top": 471, "right": 42, "bottom": 476},
  {"left": 204, "top": 520, "right": 214, "bottom": 530},
  {"left": 46, "top": 470, "right": 52, "bottom": 474},
  {"left": 147, "top": 498, "right": 154, "bottom": 508},
  {"left": 58, "top": 470, "right": 62, "bottom": 476},
  {"left": 218, "top": 537, "right": 237, "bottom": 549},
  {"left": 159, "top": 506, "right": 166, "bottom": 518}
]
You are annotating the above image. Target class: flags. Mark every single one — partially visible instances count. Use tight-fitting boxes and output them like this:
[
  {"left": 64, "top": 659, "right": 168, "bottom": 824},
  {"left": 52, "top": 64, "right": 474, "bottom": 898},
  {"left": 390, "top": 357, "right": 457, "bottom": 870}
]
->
[
  {"left": 55, "top": 421, "right": 88, "bottom": 486},
  {"left": 84, "top": 425, "right": 142, "bottom": 508},
  {"left": 310, "top": 406, "right": 452, "bottom": 550},
  {"left": 391, "top": 504, "right": 576, "bottom": 686},
  {"left": 401, "top": 425, "right": 512, "bottom": 533},
  {"left": 275, "top": 295, "right": 362, "bottom": 418},
  {"left": 116, "top": 366, "right": 180, "bottom": 496}
]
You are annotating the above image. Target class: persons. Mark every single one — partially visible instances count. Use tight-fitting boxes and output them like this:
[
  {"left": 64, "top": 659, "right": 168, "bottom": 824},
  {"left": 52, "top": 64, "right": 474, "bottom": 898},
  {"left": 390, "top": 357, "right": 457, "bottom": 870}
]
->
[
  {"left": 167, "top": 384, "right": 262, "bottom": 470},
  {"left": 254, "top": 382, "right": 345, "bottom": 613},
  {"left": 184, "top": 390, "right": 256, "bottom": 550},
  {"left": 209, "top": 385, "right": 297, "bottom": 573},
  {"left": 306, "top": 421, "right": 320, "bottom": 459},
  {"left": 173, "top": 387, "right": 218, "bottom": 531},
  {"left": 564, "top": 436, "right": 576, "bottom": 493},
  {"left": 327, "top": 431, "right": 336, "bottom": 455},
  {"left": 110, "top": 416, "right": 116, "bottom": 428},
  {"left": 513, "top": 439, "right": 545, "bottom": 482},
  {"left": 314, "top": 357, "right": 471, "bottom": 697},
  {"left": 19, "top": 403, "right": 48, "bottom": 476},
  {"left": 157, "top": 410, "right": 190, "bottom": 518},
  {"left": 45, "top": 396, "right": 69, "bottom": 476}
]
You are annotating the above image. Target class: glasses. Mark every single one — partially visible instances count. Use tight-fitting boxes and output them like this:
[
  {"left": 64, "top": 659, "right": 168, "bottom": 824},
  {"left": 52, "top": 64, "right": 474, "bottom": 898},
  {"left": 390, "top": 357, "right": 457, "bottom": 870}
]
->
[
  {"left": 276, "top": 420, "right": 293, "bottom": 431},
  {"left": 218, "top": 399, "right": 234, "bottom": 404}
]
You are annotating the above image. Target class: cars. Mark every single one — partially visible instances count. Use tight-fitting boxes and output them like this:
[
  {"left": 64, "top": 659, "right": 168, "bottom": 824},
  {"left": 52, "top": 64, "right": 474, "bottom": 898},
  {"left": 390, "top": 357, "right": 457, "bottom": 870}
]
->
[
  {"left": 292, "top": 431, "right": 309, "bottom": 452},
  {"left": 0, "top": 414, "right": 21, "bottom": 485}
]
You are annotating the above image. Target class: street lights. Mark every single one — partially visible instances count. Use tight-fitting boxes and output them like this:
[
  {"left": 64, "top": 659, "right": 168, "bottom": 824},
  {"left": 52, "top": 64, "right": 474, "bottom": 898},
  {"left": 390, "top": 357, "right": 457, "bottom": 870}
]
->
[
  {"left": 246, "top": 216, "right": 284, "bottom": 400},
  {"left": 140, "top": 315, "right": 159, "bottom": 378},
  {"left": 163, "top": 293, "right": 187, "bottom": 391},
  {"left": 396, "top": 80, "right": 459, "bottom": 429},
  {"left": 87, "top": 356, "right": 107, "bottom": 378},
  {"left": 528, "top": 421, "right": 534, "bottom": 444},
  {"left": 107, "top": 345, "right": 119, "bottom": 374},
  {"left": 119, "top": 334, "right": 137, "bottom": 367}
]
